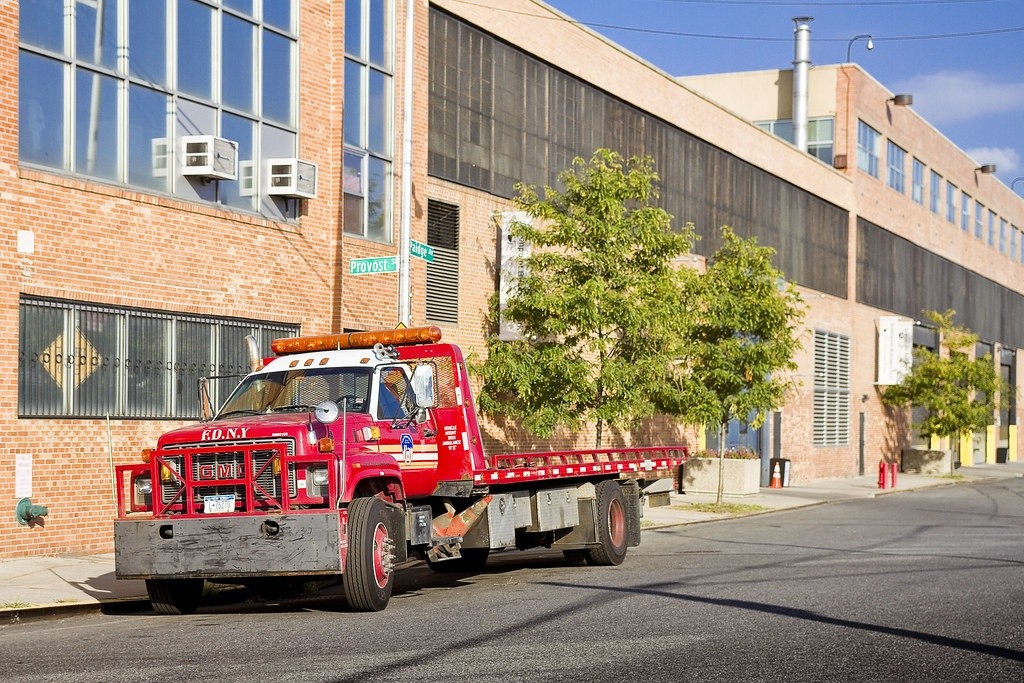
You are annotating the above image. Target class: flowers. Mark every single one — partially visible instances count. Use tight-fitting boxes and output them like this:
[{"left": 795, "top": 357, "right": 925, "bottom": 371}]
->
[{"left": 690, "top": 446, "right": 759, "bottom": 460}]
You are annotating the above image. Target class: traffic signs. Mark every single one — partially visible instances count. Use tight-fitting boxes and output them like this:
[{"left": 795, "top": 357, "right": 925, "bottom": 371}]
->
[
  {"left": 350, "top": 255, "right": 397, "bottom": 276},
  {"left": 410, "top": 237, "right": 435, "bottom": 264}
]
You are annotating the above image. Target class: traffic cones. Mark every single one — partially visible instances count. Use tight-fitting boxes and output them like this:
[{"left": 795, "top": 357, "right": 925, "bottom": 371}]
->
[{"left": 770, "top": 461, "right": 782, "bottom": 488}]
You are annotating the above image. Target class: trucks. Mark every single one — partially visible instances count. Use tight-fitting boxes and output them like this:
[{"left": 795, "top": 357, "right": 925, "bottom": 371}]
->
[{"left": 114, "top": 325, "right": 694, "bottom": 613}]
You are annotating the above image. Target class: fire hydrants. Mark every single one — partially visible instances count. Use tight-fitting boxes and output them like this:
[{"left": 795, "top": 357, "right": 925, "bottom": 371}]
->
[
  {"left": 878, "top": 460, "right": 885, "bottom": 487},
  {"left": 882, "top": 462, "right": 888, "bottom": 489},
  {"left": 891, "top": 462, "right": 897, "bottom": 487}
]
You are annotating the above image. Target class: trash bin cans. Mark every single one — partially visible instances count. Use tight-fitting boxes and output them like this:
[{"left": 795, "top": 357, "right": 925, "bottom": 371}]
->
[{"left": 769, "top": 457, "right": 791, "bottom": 487}]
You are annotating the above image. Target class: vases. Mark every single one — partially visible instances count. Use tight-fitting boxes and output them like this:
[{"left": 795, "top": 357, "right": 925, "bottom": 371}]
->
[{"left": 681, "top": 459, "right": 761, "bottom": 495}]
